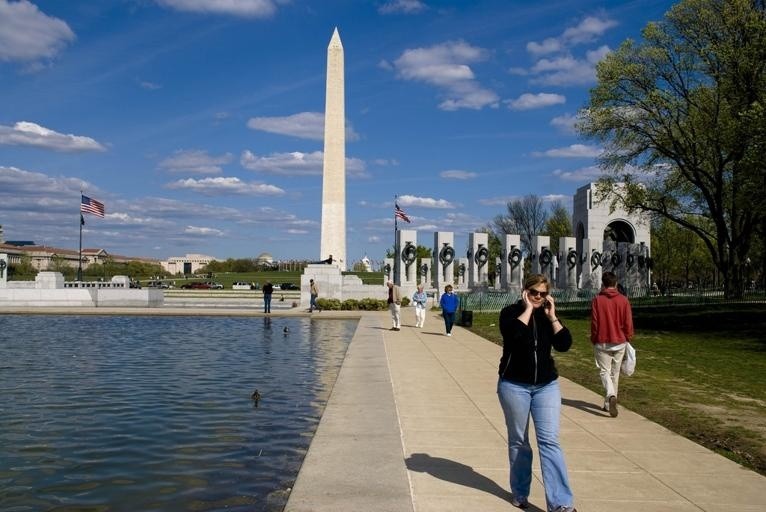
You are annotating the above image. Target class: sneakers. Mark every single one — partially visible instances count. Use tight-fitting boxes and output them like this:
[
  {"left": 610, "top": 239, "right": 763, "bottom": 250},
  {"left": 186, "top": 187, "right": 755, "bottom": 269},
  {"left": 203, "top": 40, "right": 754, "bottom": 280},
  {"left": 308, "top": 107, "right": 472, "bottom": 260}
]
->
[
  {"left": 556, "top": 505, "right": 577, "bottom": 512},
  {"left": 512, "top": 494, "right": 529, "bottom": 507},
  {"left": 608, "top": 396, "right": 618, "bottom": 417}
]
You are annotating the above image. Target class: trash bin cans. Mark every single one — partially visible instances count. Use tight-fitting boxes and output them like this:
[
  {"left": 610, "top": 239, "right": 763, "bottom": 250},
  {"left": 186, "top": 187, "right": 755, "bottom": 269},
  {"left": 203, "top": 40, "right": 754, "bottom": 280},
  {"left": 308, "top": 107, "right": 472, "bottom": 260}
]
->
[{"left": 462, "top": 310, "right": 473, "bottom": 327}]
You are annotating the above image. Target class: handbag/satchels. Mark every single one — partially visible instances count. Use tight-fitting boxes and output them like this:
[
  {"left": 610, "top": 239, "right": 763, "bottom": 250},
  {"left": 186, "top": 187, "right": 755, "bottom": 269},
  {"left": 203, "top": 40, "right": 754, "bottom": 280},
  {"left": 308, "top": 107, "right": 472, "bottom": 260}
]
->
[{"left": 620, "top": 342, "right": 636, "bottom": 377}]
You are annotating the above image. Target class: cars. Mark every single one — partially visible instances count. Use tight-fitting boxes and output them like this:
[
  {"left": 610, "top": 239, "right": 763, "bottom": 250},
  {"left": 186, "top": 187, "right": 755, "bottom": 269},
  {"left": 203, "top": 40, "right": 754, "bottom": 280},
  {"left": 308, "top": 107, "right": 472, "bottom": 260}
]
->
[
  {"left": 181, "top": 281, "right": 224, "bottom": 289},
  {"left": 272, "top": 283, "right": 301, "bottom": 290}
]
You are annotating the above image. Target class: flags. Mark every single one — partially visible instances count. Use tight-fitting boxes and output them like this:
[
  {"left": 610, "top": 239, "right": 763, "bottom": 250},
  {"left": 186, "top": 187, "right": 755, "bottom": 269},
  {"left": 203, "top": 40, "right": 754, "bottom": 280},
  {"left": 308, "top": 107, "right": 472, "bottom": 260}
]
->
[
  {"left": 80, "top": 195, "right": 105, "bottom": 219},
  {"left": 394, "top": 203, "right": 411, "bottom": 224}
]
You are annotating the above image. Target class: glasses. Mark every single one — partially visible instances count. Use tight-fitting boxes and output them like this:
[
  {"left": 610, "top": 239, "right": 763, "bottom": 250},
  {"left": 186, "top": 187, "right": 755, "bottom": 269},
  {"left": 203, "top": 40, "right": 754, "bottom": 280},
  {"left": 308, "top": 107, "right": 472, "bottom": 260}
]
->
[{"left": 529, "top": 288, "right": 549, "bottom": 298}]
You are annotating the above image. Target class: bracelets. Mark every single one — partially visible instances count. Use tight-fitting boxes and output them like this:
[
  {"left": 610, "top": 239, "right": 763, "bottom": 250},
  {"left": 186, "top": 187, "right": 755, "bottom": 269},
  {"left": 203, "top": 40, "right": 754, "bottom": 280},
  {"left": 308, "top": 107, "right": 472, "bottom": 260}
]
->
[{"left": 551, "top": 318, "right": 559, "bottom": 323}]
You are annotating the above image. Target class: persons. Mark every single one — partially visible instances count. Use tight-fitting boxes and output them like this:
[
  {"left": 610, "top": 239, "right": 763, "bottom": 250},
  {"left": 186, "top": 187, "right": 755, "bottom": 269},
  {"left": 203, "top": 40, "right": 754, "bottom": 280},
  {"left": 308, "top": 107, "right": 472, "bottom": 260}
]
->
[
  {"left": 307, "top": 279, "right": 323, "bottom": 313},
  {"left": 261, "top": 316, "right": 273, "bottom": 354},
  {"left": 495, "top": 272, "right": 580, "bottom": 511},
  {"left": 412, "top": 284, "right": 428, "bottom": 328},
  {"left": 310, "top": 254, "right": 333, "bottom": 264},
  {"left": 590, "top": 271, "right": 636, "bottom": 418},
  {"left": 386, "top": 280, "right": 402, "bottom": 331},
  {"left": 439, "top": 285, "right": 460, "bottom": 337},
  {"left": 651, "top": 282, "right": 659, "bottom": 297},
  {"left": 262, "top": 279, "right": 273, "bottom": 314}
]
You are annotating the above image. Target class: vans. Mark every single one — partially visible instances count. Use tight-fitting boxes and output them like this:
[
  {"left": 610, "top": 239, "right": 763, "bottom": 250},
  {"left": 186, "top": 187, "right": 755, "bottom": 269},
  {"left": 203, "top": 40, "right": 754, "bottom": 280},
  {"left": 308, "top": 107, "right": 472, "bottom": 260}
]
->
[{"left": 232, "top": 281, "right": 251, "bottom": 290}]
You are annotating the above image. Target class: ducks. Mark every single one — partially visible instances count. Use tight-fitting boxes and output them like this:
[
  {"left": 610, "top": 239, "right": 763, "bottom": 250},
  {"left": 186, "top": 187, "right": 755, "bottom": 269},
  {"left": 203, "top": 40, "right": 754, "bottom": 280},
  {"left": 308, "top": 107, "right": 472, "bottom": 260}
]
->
[
  {"left": 283, "top": 326, "right": 289, "bottom": 332},
  {"left": 251, "top": 389, "right": 261, "bottom": 400}
]
